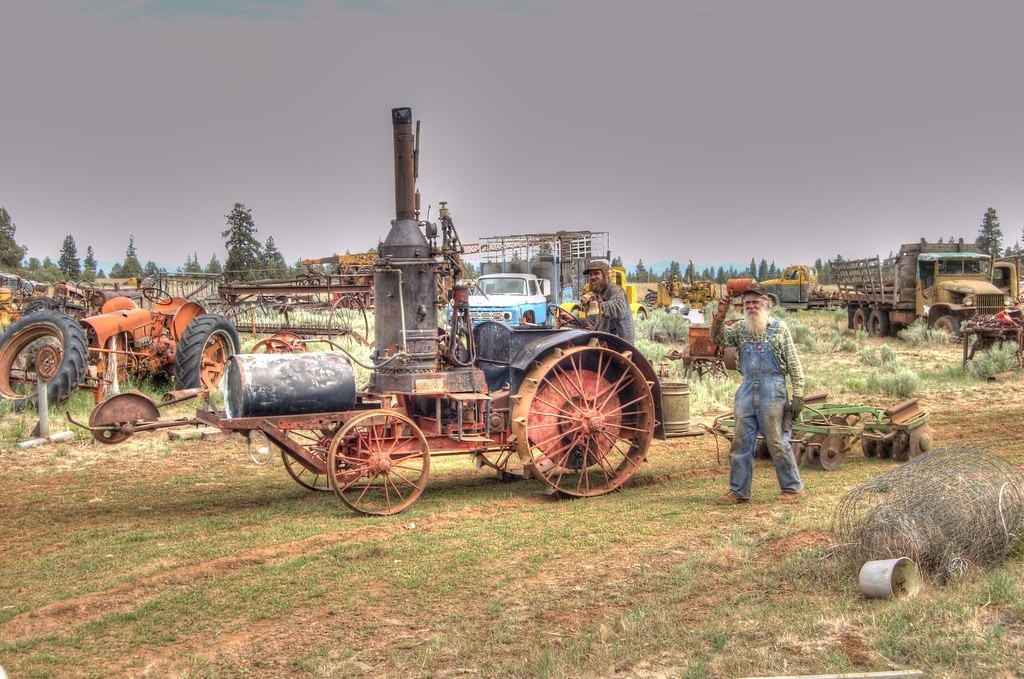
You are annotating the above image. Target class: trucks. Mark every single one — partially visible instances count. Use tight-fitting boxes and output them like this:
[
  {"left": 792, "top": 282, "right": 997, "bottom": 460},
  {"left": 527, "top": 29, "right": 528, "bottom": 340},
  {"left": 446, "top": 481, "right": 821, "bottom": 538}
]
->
[
  {"left": 447, "top": 231, "right": 611, "bottom": 335},
  {"left": 758, "top": 265, "right": 841, "bottom": 311},
  {"left": 827, "top": 236, "right": 1005, "bottom": 338}
]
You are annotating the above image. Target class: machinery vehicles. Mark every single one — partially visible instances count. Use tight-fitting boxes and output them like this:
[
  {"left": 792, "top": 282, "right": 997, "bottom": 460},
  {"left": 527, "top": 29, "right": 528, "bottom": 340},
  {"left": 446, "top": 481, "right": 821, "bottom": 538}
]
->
[
  {"left": 195, "top": 107, "right": 669, "bottom": 516},
  {"left": 644, "top": 260, "right": 720, "bottom": 313},
  {"left": 556, "top": 267, "right": 647, "bottom": 326}
]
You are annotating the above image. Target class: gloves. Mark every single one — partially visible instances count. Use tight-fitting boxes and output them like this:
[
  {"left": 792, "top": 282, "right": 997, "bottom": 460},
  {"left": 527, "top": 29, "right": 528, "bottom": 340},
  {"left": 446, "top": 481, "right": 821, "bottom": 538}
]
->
[{"left": 783, "top": 395, "right": 804, "bottom": 432}]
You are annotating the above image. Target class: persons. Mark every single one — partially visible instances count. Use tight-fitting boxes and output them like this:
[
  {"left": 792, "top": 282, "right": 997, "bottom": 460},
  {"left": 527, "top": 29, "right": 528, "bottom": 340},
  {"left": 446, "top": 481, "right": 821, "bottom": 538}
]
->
[
  {"left": 708, "top": 281, "right": 806, "bottom": 507},
  {"left": 553, "top": 260, "right": 649, "bottom": 462}
]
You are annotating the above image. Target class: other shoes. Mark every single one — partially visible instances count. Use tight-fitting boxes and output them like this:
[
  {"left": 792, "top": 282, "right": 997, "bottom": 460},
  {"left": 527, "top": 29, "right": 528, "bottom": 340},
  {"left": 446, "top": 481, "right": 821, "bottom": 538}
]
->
[
  {"left": 775, "top": 489, "right": 805, "bottom": 500},
  {"left": 715, "top": 492, "right": 750, "bottom": 505}
]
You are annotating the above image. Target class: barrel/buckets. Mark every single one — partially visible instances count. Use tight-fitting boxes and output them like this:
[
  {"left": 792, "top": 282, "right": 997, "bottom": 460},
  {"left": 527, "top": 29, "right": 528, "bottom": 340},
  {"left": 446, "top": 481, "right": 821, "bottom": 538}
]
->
[
  {"left": 221, "top": 351, "right": 355, "bottom": 415},
  {"left": 860, "top": 556, "right": 920, "bottom": 601},
  {"left": 660, "top": 382, "right": 690, "bottom": 432}
]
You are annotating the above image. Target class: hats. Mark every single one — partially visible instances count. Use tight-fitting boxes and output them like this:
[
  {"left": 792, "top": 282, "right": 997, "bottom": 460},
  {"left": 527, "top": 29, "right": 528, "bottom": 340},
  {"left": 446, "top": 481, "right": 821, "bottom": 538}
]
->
[
  {"left": 583, "top": 261, "right": 609, "bottom": 275},
  {"left": 742, "top": 283, "right": 768, "bottom": 295}
]
